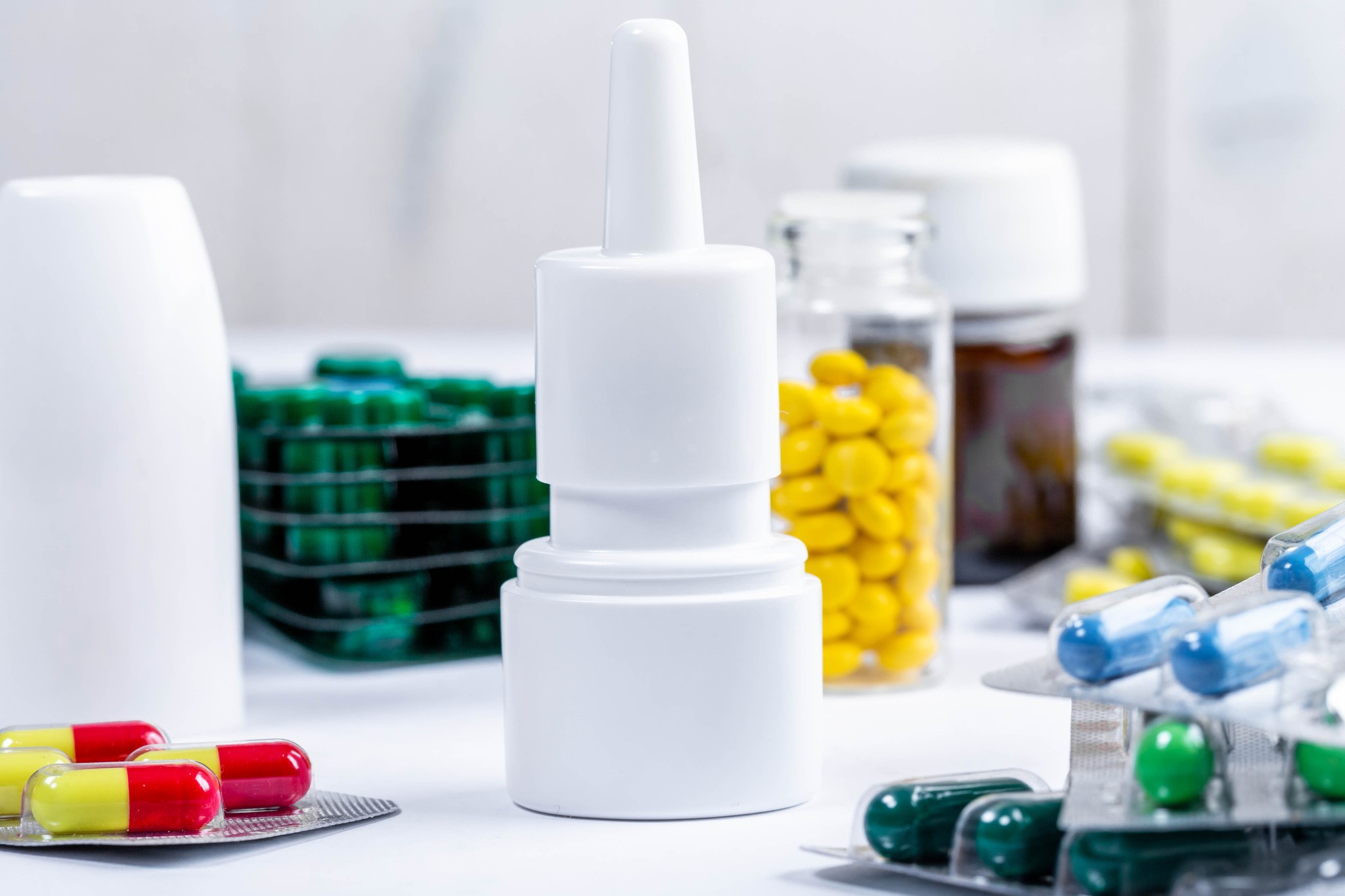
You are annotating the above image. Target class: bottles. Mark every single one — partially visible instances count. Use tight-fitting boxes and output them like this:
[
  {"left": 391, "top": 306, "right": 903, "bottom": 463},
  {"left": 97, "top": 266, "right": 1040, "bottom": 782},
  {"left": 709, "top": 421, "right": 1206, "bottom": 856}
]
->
[
  {"left": 842, "top": 137, "right": 1083, "bottom": 589},
  {"left": 501, "top": 15, "right": 825, "bottom": 818},
  {"left": 763, "top": 189, "right": 954, "bottom": 700}
]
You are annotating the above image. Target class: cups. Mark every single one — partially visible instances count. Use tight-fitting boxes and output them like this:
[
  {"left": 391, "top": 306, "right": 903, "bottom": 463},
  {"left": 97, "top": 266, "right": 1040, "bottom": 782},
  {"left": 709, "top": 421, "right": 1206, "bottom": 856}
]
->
[{"left": 0, "top": 174, "right": 241, "bottom": 745}]
